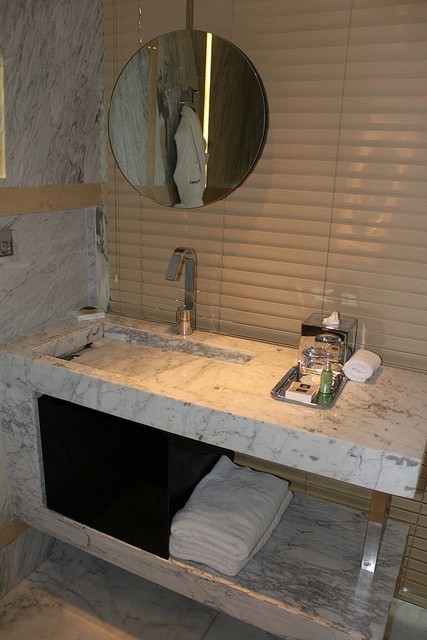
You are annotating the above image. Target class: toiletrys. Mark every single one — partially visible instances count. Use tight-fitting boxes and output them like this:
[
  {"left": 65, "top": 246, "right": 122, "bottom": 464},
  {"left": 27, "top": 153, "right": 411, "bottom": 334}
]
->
[{"left": 319, "top": 359, "right": 334, "bottom": 394}]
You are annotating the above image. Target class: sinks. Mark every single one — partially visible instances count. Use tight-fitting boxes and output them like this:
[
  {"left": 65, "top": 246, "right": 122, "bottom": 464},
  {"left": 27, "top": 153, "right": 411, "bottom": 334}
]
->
[{"left": 31, "top": 323, "right": 252, "bottom": 391}]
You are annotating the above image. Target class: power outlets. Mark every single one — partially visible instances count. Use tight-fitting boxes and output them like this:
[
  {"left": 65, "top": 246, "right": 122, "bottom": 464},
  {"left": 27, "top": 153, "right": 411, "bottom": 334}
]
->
[{"left": 0, "top": 227, "right": 15, "bottom": 258}]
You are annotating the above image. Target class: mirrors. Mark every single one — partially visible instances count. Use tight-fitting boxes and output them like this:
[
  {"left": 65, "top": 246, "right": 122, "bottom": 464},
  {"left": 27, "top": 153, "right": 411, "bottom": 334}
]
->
[{"left": 108, "top": 29, "right": 267, "bottom": 209}]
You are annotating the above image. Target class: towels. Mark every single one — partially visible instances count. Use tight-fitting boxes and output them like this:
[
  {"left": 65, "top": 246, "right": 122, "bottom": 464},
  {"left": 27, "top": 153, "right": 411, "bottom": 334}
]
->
[
  {"left": 173, "top": 104, "right": 210, "bottom": 207},
  {"left": 168, "top": 454, "right": 295, "bottom": 578},
  {"left": 342, "top": 349, "right": 382, "bottom": 383}
]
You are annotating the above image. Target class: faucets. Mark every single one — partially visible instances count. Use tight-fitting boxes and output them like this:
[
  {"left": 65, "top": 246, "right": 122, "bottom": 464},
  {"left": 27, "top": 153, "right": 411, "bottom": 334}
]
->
[{"left": 165, "top": 247, "right": 197, "bottom": 335}]
